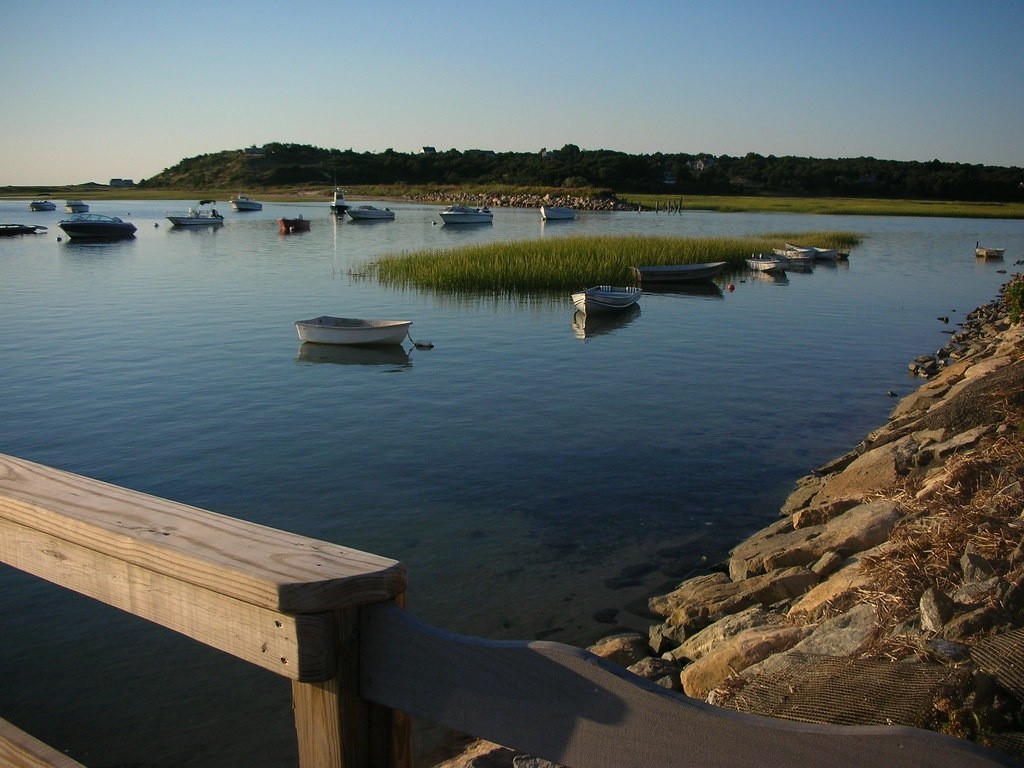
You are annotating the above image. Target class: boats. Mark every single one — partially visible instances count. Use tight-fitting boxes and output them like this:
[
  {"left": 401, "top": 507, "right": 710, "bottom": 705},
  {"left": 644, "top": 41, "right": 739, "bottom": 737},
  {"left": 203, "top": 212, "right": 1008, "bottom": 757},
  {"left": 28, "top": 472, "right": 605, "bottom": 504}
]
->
[
  {"left": 57, "top": 213, "right": 137, "bottom": 238},
  {"left": 344, "top": 205, "right": 395, "bottom": 219},
  {"left": 539, "top": 206, "right": 576, "bottom": 220},
  {"left": 786, "top": 242, "right": 839, "bottom": 259},
  {"left": 27, "top": 201, "right": 56, "bottom": 212},
  {"left": 976, "top": 257, "right": 1005, "bottom": 264},
  {"left": 975, "top": 240, "right": 1006, "bottom": 257},
  {"left": 438, "top": 206, "right": 493, "bottom": 224},
  {"left": 64, "top": 200, "right": 90, "bottom": 213},
  {"left": 293, "top": 315, "right": 413, "bottom": 345},
  {"left": 749, "top": 270, "right": 790, "bottom": 286},
  {"left": 0, "top": 224, "right": 36, "bottom": 234},
  {"left": 329, "top": 188, "right": 352, "bottom": 213},
  {"left": 636, "top": 279, "right": 725, "bottom": 299},
  {"left": 166, "top": 207, "right": 224, "bottom": 225},
  {"left": 278, "top": 214, "right": 311, "bottom": 235},
  {"left": 745, "top": 253, "right": 789, "bottom": 271},
  {"left": 772, "top": 248, "right": 817, "bottom": 260},
  {"left": 229, "top": 195, "right": 262, "bottom": 211},
  {"left": 634, "top": 262, "right": 727, "bottom": 282},
  {"left": 571, "top": 286, "right": 643, "bottom": 316},
  {"left": 168, "top": 225, "right": 224, "bottom": 233},
  {"left": 297, "top": 341, "right": 412, "bottom": 366},
  {"left": 572, "top": 303, "right": 641, "bottom": 339}
]
